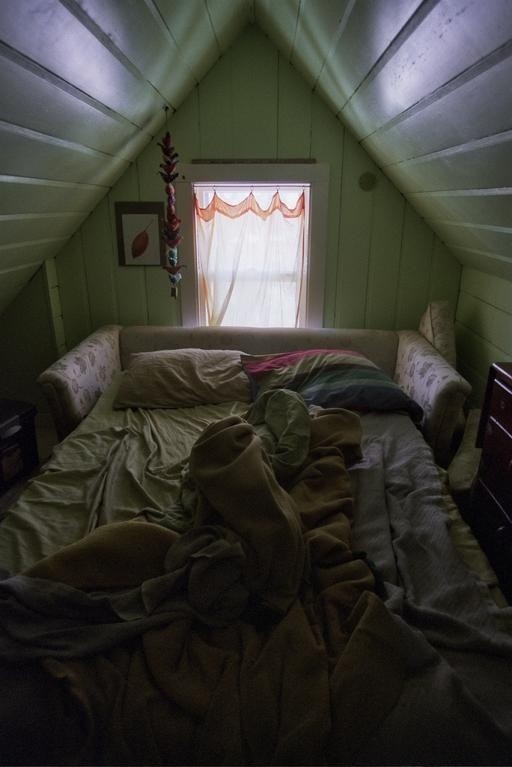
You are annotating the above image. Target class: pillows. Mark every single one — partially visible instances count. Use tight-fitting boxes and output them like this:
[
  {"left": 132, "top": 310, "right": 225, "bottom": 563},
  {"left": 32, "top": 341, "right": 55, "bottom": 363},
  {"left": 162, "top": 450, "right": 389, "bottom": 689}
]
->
[
  {"left": 109, "top": 347, "right": 254, "bottom": 411},
  {"left": 237, "top": 347, "right": 424, "bottom": 425}
]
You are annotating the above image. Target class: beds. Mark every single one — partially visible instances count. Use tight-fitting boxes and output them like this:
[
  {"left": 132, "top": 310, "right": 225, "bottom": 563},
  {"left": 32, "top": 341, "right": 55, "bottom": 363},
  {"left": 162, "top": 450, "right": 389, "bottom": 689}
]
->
[{"left": 0, "top": 322, "right": 512, "bottom": 766}]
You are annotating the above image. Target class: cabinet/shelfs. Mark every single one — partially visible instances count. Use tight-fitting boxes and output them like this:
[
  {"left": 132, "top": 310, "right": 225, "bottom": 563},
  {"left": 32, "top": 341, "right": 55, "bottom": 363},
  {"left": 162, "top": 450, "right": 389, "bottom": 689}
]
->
[
  {"left": 0, "top": 396, "right": 40, "bottom": 501},
  {"left": 466, "top": 361, "right": 512, "bottom": 608}
]
